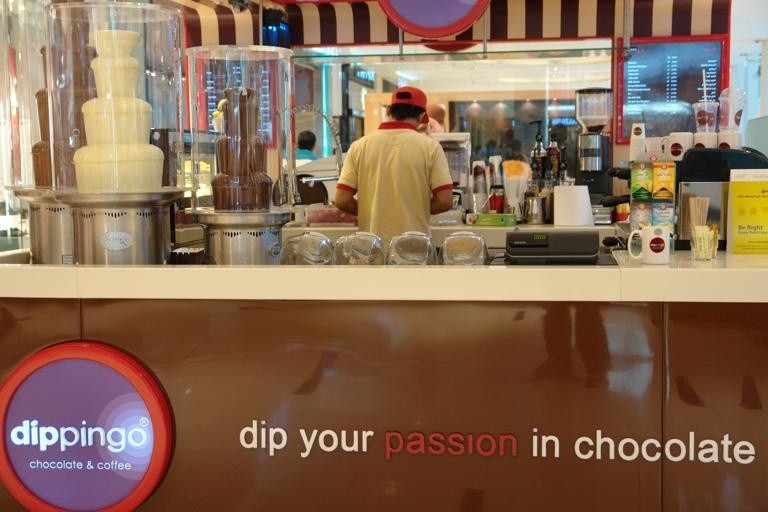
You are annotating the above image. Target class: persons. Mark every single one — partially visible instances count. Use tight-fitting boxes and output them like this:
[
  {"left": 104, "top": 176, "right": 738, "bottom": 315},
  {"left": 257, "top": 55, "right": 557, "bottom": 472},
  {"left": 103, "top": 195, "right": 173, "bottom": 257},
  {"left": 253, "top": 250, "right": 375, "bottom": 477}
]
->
[
  {"left": 278, "top": 129, "right": 323, "bottom": 163},
  {"left": 336, "top": 90, "right": 455, "bottom": 247},
  {"left": 471, "top": 130, "right": 527, "bottom": 162}
]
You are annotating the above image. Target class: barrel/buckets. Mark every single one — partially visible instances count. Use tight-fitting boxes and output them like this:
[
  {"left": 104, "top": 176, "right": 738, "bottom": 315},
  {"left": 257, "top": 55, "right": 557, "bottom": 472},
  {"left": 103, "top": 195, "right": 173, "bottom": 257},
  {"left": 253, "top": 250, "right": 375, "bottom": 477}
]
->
[{"left": 575, "top": 88, "right": 613, "bottom": 132}]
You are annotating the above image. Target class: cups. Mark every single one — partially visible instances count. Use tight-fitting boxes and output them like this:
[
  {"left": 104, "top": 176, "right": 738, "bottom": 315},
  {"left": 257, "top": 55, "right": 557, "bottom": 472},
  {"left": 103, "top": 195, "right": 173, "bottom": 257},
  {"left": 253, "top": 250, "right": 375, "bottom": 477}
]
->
[
  {"left": 626, "top": 223, "right": 671, "bottom": 265},
  {"left": 689, "top": 222, "right": 719, "bottom": 262},
  {"left": 718, "top": 85, "right": 748, "bottom": 130},
  {"left": 519, "top": 195, "right": 546, "bottom": 226},
  {"left": 718, "top": 132, "right": 743, "bottom": 152},
  {"left": 627, "top": 122, "right": 647, "bottom": 163},
  {"left": 469, "top": 154, "right": 506, "bottom": 214},
  {"left": 659, "top": 131, "right": 693, "bottom": 161},
  {"left": 692, "top": 131, "right": 716, "bottom": 151},
  {"left": 690, "top": 102, "right": 719, "bottom": 132}
]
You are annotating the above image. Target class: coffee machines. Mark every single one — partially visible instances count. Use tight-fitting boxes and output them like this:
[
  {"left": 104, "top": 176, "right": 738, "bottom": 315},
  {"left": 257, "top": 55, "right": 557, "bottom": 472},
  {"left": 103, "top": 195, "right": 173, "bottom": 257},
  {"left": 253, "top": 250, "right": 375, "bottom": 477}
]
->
[{"left": 570, "top": 85, "right": 616, "bottom": 200}]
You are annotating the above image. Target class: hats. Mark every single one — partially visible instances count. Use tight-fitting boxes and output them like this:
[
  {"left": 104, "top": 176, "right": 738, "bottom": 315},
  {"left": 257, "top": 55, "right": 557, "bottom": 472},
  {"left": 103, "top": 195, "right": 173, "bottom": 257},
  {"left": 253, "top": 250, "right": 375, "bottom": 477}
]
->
[{"left": 391, "top": 86, "right": 429, "bottom": 124}]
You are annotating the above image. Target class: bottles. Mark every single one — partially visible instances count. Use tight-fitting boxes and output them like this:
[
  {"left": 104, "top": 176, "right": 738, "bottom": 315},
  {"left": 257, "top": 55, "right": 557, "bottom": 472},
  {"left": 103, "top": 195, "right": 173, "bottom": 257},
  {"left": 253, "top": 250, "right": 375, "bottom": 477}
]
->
[
  {"left": 334, "top": 231, "right": 386, "bottom": 265},
  {"left": 631, "top": 187, "right": 652, "bottom": 229},
  {"left": 438, "top": 230, "right": 490, "bottom": 266},
  {"left": 546, "top": 130, "right": 562, "bottom": 182},
  {"left": 279, "top": 229, "right": 334, "bottom": 266},
  {"left": 652, "top": 187, "right": 675, "bottom": 235},
  {"left": 387, "top": 230, "right": 438, "bottom": 265}
]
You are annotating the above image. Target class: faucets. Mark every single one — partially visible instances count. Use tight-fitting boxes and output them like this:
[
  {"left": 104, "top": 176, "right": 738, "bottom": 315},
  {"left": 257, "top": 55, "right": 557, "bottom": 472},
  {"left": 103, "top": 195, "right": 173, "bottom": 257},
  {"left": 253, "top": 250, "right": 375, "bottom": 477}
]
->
[{"left": 292, "top": 102, "right": 344, "bottom": 176}]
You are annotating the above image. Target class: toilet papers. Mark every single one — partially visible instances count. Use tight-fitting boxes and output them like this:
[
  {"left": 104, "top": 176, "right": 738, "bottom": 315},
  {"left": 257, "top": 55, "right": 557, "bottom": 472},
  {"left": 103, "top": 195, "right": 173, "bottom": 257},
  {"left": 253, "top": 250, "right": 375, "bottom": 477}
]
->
[{"left": 553, "top": 184, "right": 595, "bottom": 227}]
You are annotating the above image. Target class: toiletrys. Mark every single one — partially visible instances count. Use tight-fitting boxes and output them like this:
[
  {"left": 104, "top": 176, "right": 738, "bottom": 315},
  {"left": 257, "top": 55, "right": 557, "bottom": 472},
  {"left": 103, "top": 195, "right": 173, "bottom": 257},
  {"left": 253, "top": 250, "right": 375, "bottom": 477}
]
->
[
  {"left": 528, "top": 119, "right": 547, "bottom": 179},
  {"left": 547, "top": 132, "right": 562, "bottom": 179}
]
